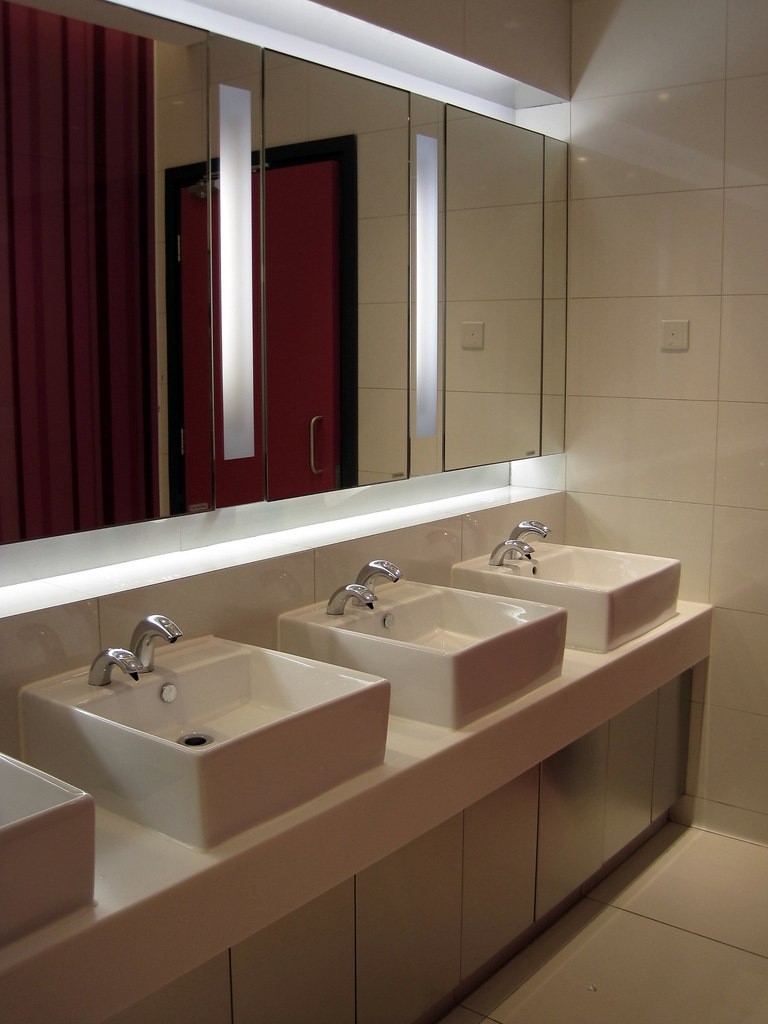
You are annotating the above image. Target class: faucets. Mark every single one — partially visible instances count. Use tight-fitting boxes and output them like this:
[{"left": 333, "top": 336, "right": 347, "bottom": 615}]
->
[
  {"left": 348, "top": 557, "right": 403, "bottom": 606},
  {"left": 129, "top": 613, "right": 182, "bottom": 673},
  {"left": 322, "top": 582, "right": 379, "bottom": 616},
  {"left": 505, "top": 519, "right": 553, "bottom": 559},
  {"left": 488, "top": 538, "right": 536, "bottom": 567},
  {"left": 84, "top": 642, "right": 147, "bottom": 688}
]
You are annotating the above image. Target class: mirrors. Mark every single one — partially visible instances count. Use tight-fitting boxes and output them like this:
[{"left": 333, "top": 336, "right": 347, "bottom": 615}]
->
[{"left": 0, "top": 0, "right": 571, "bottom": 546}]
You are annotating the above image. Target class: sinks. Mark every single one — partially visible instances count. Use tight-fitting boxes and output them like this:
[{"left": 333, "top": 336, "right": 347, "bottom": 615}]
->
[
  {"left": 0, "top": 749, "right": 97, "bottom": 950},
  {"left": 15, "top": 633, "right": 395, "bottom": 855},
  {"left": 275, "top": 576, "right": 571, "bottom": 736},
  {"left": 450, "top": 537, "right": 682, "bottom": 656}
]
